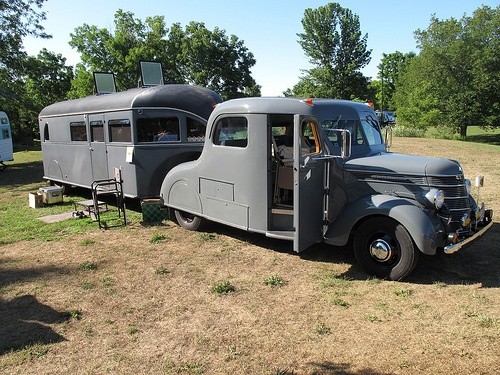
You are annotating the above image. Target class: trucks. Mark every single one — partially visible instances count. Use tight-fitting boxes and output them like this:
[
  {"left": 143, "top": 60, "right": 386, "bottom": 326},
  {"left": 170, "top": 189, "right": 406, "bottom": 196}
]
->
[{"left": 38, "top": 84, "right": 224, "bottom": 210}]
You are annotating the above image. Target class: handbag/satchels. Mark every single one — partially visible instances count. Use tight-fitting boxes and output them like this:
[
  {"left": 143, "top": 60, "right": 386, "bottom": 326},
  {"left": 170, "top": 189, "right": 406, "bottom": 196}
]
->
[{"left": 277, "top": 145, "right": 311, "bottom": 167}]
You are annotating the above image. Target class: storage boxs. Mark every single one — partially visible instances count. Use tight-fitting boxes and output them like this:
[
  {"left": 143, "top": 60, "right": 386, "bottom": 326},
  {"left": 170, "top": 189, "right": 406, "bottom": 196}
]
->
[
  {"left": 29, "top": 192, "right": 43, "bottom": 208},
  {"left": 40, "top": 186, "right": 63, "bottom": 206}
]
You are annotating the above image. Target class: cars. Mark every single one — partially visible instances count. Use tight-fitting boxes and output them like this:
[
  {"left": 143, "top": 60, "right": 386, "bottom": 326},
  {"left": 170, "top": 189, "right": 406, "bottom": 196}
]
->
[
  {"left": 386, "top": 111, "right": 395, "bottom": 122},
  {"left": 159, "top": 96, "right": 494, "bottom": 282}
]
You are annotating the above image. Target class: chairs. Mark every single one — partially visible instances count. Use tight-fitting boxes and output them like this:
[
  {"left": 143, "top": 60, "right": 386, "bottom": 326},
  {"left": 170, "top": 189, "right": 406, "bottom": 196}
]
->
[{"left": 91, "top": 168, "right": 126, "bottom": 229}]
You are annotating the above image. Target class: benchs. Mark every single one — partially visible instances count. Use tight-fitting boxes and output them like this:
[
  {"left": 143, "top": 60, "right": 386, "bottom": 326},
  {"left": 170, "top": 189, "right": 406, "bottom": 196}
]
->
[{"left": 220, "top": 134, "right": 314, "bottom": 207}]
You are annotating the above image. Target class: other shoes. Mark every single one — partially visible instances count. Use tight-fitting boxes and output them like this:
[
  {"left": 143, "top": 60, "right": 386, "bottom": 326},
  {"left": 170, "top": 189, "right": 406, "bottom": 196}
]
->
[{"left": 73, "top": 210, "right": 87, "bottom": 219}]
